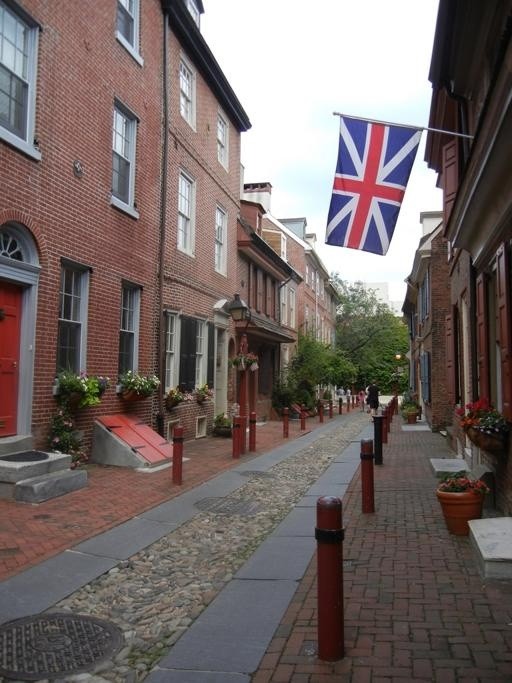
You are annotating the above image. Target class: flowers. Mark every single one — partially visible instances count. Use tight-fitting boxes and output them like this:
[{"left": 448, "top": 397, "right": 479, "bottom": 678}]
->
[
  {"left": 59, "top": 373, "right": 110, "bottom": 408},
  {"left": 457, "top": 398, "right": 512, "bottom": 435},
  {"left": 196, "top": 385, "right": 214, "bottom": 400},
  {"left": 437, "top": 471, "right": 492, "bottom": 496},
  {"left": 228, "top": 352, "right": 259, "bottom": 371},
  {"left": 168, "top": 389, "right": 194, "bottom": 402},
  {"left": 119, "top": 370, "right": 161, "bottom": 396}
]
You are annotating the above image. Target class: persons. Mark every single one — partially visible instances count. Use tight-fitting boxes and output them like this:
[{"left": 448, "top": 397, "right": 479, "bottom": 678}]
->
[{"left": 366, "top": 378, "right": 381, "bottom": 422}]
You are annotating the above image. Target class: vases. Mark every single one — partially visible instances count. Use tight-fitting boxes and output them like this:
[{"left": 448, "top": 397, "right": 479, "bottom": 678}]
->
[
  {"left": 61, "top": 393, "right": 87, "bottom": 405},
  {"left": 235, "top": 363, "right": 248, "bottom": 372},
  {"left": 165, "top": 400, "right": 181, "bottom": 410},
  {"left": 466, "top": 426, "right": 505, "bottom": 452},
  {"left": 197, "top": 397, "right": 206, "bottom": 404},
  {"left": 435, "top": 488, "right": 485, "bottom": 534},
  {"left": 121, "top": 389, "right": 148, "bottom": 402}
]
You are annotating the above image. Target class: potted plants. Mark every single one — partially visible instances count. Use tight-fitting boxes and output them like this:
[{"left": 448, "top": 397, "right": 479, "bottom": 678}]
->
[
  {"left": 407, "top": 406, "right": 418, "bottom": 423},
  {"left": 214, "top": 412, "right": 232, "bottom": 436}
]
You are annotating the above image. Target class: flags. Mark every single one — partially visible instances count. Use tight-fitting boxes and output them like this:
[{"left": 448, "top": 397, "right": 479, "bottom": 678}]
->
[{"left": 323, "top": 116, "right": 424, "bottom": 257}]
